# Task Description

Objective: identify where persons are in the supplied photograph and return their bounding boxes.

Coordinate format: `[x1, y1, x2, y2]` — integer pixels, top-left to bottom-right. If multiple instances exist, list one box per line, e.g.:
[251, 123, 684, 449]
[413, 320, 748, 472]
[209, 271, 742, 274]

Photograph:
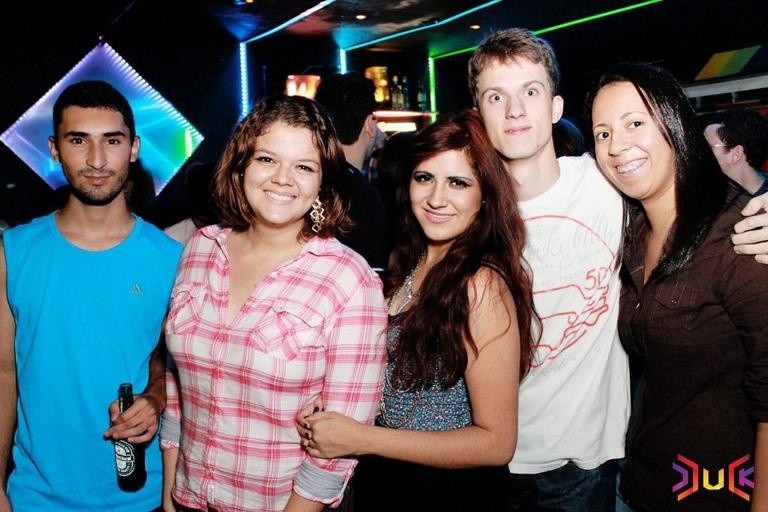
[292, 108, 545, 512]
[159, 93, 390, 512]
[465, 27, 768, 512]
[580, 58, 768, 512]
[319, 71, 394, 273]
[0, 79, 185, 512]
[699, 103, 768, 199]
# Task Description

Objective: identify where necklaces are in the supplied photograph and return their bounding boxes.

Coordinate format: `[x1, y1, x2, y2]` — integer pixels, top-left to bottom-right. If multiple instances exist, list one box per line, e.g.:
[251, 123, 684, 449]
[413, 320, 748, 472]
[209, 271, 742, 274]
[393, 254, 425, 313]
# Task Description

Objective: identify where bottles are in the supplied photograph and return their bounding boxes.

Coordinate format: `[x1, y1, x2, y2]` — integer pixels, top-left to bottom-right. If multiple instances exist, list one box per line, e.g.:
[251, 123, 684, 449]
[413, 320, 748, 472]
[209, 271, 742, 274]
[390, 74, 410, 109]
[113, 382, 147, 493]
[416, 79, 427, 111]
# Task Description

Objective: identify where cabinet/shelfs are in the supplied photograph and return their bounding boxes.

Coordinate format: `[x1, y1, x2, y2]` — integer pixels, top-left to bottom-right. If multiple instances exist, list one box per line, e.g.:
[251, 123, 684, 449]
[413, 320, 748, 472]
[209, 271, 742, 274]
[371, 110, 441, 138]
[680, 73, 768, 127]
